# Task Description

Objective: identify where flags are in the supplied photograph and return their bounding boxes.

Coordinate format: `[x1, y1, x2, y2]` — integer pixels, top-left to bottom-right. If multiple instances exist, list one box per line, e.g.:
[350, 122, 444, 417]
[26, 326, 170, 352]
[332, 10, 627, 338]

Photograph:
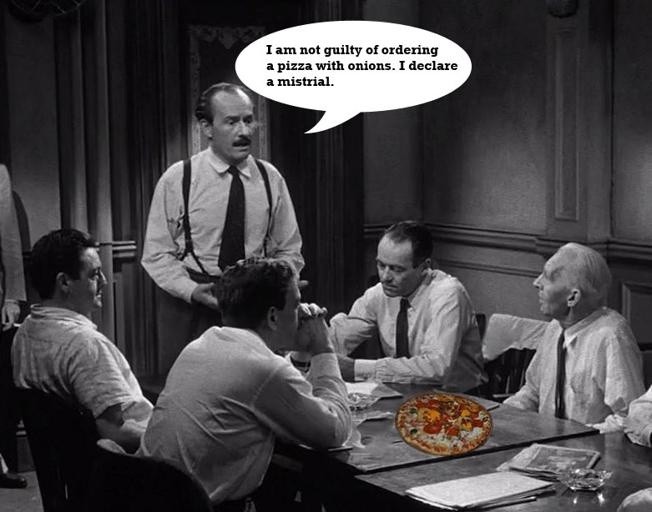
[4, 321, 24, 328]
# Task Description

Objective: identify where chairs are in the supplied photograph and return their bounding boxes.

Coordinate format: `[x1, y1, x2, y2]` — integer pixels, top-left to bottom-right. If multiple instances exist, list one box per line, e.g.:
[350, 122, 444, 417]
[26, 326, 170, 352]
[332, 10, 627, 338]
[17, 388, 213, 510]
[483, 313, 551, 400]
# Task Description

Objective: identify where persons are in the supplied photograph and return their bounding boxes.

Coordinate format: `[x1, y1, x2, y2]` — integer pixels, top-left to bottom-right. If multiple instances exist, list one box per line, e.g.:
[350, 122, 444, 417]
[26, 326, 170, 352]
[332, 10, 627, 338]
[498, 234, 633, 436]
[0, 158, 35, 490]
[326, 218, 491, 396]
[9, 226, 158, 457]
[621, 382, 651, 448]
[136, 80, 304, 384]
[125, 248, 353, 505]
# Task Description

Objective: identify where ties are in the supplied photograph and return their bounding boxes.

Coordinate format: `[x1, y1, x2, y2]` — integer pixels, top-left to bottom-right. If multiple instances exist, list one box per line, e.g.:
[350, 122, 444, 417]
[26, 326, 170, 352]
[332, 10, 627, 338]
[555, 334, 566, 418]
[394, 298, 411, 358]
[217, 166, 246, 272]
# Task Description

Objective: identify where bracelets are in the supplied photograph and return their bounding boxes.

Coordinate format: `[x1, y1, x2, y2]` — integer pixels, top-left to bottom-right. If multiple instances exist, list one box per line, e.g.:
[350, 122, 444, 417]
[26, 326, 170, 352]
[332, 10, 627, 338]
[289, 354, 313, 371]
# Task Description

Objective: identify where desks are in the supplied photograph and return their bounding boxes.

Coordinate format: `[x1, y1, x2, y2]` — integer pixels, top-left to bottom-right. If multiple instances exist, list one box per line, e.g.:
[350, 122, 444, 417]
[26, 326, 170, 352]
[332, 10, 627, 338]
[332, 383, 652, 511]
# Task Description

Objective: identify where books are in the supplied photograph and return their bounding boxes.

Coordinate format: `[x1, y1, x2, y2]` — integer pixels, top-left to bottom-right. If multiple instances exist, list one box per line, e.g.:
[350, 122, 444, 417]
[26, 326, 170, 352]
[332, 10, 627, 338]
[403, 440, 603, 509]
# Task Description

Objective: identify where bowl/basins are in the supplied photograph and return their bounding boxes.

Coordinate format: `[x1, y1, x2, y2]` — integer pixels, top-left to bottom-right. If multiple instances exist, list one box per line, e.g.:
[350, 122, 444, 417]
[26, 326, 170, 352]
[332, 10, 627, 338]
[557, 468, 614, 492]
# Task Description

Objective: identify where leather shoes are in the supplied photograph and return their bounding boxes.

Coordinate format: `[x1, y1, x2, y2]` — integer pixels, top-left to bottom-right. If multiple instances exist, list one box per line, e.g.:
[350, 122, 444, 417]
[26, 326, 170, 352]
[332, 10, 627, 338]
[0, 471, 27, 488]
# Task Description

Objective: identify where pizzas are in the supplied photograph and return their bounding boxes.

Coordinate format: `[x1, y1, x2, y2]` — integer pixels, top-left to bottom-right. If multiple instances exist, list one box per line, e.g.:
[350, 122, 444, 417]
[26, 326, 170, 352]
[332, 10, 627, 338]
[397, 392, 492, 455]
